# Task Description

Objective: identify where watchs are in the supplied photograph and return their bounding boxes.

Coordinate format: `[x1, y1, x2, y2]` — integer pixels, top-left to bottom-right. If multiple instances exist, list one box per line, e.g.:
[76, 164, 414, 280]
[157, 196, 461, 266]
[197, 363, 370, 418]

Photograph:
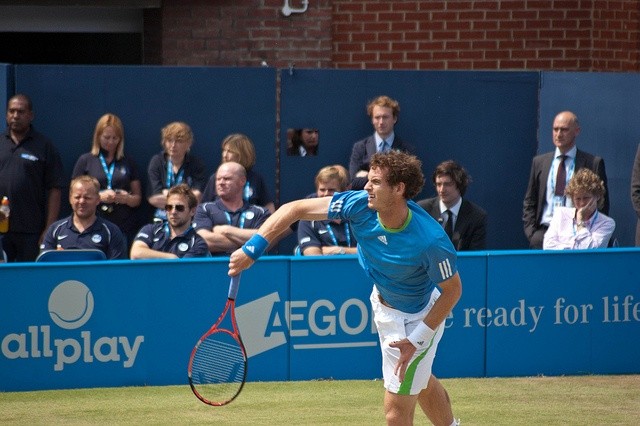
[338, 246, 346, 255]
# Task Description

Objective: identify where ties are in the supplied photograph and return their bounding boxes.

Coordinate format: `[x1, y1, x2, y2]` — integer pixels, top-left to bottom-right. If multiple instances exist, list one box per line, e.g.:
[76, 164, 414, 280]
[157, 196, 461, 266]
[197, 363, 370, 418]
[444, 209, 454, 242]
[379, 140, 388, 152]
[554, 154, 570, 197]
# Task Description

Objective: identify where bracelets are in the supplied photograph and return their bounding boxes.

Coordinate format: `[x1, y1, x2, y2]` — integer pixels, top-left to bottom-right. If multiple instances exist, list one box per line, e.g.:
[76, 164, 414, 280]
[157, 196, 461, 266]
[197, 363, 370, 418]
[406, 319, 437, 352]
[240, 232, 270, 263]
[577, 220, 591, 226]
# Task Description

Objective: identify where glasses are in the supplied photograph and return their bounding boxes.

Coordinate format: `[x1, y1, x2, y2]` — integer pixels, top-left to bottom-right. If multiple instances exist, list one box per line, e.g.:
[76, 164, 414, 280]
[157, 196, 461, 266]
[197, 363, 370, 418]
[165, 205, 186, 212]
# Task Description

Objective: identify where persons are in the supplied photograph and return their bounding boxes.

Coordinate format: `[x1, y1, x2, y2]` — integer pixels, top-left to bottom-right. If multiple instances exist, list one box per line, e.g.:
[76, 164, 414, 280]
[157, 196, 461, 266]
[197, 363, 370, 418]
[298, 163, 358, 257]
[190, 160, 274, 257]
[1, 92, 65, 261]
[630, 141, 639, 247]
[70, 112, 143, 257]
[520, 110, 611, 250]
[227, 148, 464, 426]
[146, 121, 208, 225]
[36, 174, 129, 262]
[127, 184, 213, 260]
[542, 167, 618, 250]
[414, 159, 489, 252]
[289, 127, 318, 156]
[198, 133, 277, 216]
[349, 95, 419, 191]
